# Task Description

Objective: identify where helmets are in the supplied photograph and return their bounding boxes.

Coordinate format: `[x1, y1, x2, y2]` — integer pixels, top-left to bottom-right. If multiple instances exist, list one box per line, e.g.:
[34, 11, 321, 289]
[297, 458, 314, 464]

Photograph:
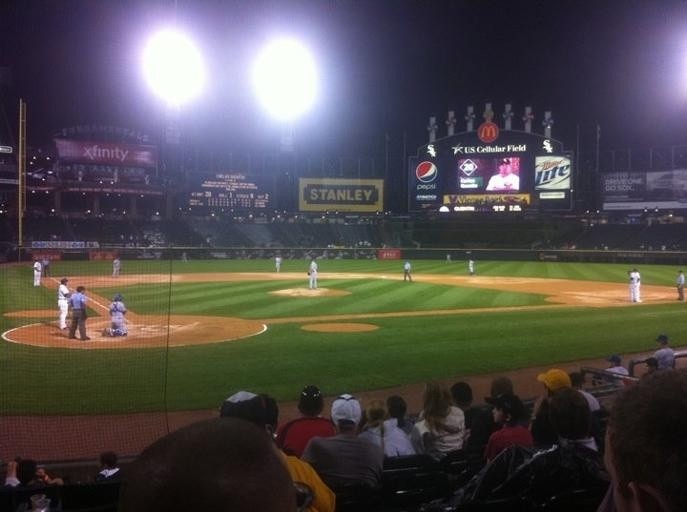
[331, 394, 361, 428]
[298, 384, 323, 416]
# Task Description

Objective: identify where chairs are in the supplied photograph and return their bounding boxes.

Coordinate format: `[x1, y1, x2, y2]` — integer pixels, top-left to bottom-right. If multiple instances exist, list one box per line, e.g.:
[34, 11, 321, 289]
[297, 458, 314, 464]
[277, 393, 618, 508]
[6, 455, 120, 504]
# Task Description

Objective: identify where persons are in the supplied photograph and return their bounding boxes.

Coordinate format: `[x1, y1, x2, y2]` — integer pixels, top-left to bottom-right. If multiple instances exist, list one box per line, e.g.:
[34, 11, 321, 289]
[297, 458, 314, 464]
[634, 268, 642, 302]
[484, 157, 520, 191]
[275, 254, 283, 274]
[33, 253, 128, 342]
[445, 253, 452, 265]
[628, 270, 635, 302]
[1, 332, 687, 511]
[468, 258, 475, 277]
[182, 251, 187, 264]
[307, 255, 318, 290]
[675, 270, 685, 301]
[403, 260, 412, 282]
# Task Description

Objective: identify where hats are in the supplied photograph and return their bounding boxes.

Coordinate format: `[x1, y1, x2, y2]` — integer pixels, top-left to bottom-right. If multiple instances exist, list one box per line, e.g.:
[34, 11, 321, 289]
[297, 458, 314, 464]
[655, 335, 668, 346]
[537, 368, 572, 393]
[485, 393, 522, 418]
[220, 390, 278, 432]
[605, 354, 622, 366]
